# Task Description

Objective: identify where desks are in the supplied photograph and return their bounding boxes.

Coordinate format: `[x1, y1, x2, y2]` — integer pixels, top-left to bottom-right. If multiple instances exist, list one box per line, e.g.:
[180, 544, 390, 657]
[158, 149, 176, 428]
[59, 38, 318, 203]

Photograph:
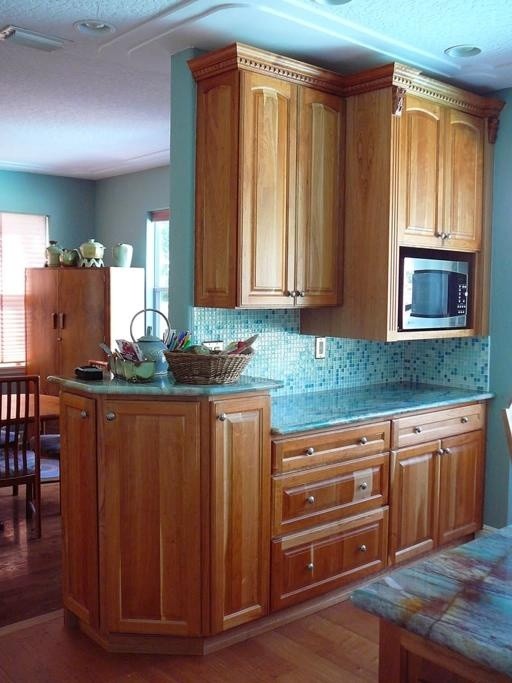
[0, 393, 60, 435]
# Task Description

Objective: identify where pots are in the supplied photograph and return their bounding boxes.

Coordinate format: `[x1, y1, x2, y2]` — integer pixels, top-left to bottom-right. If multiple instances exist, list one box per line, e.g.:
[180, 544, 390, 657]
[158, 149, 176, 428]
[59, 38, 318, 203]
[79, 238, 104, 259]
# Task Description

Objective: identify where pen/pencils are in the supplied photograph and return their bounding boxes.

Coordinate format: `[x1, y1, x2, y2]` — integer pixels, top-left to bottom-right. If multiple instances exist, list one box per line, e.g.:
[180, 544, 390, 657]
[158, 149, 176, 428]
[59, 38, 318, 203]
[169, 331, 191, 351]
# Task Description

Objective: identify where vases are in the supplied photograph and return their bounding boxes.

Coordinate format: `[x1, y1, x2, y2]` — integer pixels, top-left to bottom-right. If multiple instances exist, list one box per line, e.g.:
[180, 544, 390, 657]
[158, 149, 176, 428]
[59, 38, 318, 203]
[113, 243, 133, 267]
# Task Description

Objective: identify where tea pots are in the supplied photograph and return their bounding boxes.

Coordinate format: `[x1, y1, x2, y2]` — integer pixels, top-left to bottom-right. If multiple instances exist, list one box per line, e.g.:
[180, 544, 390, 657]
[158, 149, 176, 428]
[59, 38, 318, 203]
[129, 308, 171, 374]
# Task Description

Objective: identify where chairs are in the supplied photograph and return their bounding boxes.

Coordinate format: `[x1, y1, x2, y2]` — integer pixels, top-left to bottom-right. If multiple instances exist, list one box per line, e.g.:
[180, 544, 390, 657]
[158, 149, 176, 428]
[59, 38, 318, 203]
[0, 376, 60, 539]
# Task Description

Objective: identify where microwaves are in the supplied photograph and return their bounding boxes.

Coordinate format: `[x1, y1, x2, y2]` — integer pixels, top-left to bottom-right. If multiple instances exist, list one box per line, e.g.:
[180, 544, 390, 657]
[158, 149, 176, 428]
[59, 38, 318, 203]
[401, 257, 470, 329]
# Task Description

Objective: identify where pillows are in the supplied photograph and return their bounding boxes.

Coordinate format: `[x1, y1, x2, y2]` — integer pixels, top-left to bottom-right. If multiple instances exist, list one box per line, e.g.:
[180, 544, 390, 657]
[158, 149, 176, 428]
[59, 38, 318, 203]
[0, 448, 35, 478]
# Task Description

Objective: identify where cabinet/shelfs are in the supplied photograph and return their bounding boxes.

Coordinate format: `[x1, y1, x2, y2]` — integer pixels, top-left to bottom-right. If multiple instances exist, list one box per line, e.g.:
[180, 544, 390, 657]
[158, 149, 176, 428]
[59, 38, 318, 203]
[204, 391, 265, 657]
[391, 400, 488, 576]
[299, 62, 506, 343]
[187, 42, 345, 310]
[272, 419, 390, 629]
[57, 391, 204, 657]
[24, 267, 145, 398]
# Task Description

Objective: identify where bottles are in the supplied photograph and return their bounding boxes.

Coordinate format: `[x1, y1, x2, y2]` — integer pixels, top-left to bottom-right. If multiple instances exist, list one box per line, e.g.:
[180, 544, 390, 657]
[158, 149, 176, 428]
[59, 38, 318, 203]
[45, 240, 64, 267]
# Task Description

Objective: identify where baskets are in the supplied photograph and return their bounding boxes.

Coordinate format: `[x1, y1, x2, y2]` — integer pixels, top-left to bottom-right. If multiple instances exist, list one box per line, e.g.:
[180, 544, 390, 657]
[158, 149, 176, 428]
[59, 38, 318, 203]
[165, 346, 255, 384]
